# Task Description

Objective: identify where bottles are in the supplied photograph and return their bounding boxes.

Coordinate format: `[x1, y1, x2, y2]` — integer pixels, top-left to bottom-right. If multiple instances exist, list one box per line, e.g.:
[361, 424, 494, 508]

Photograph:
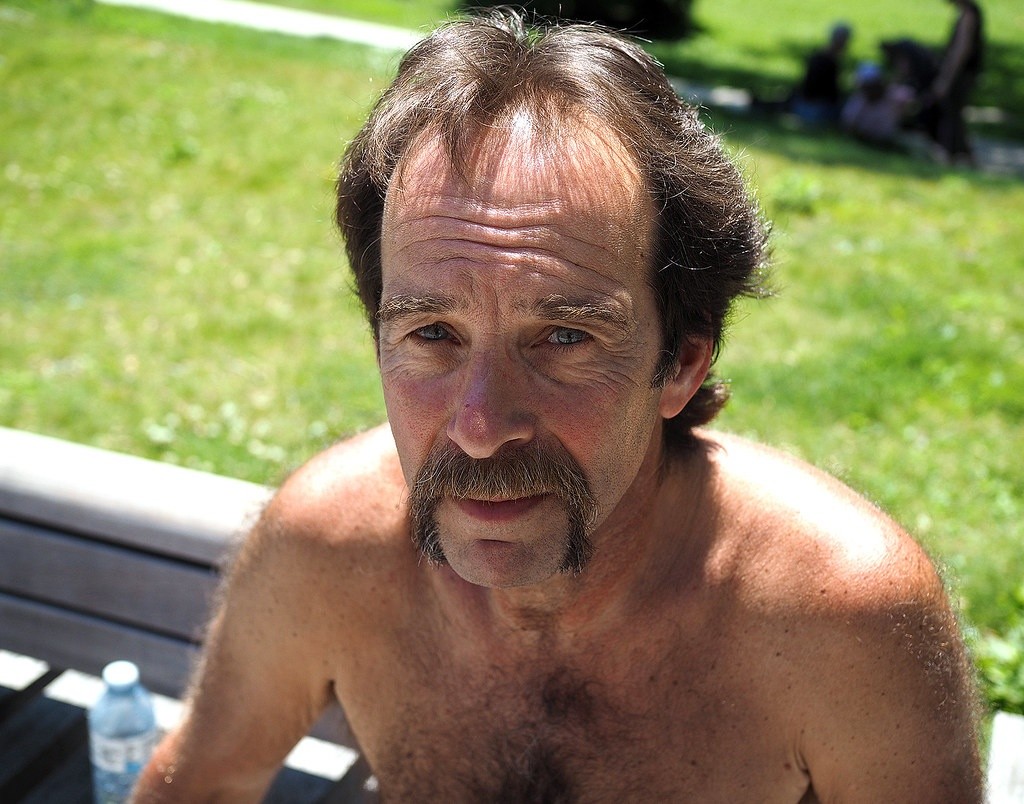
[87, 660, 158, 804]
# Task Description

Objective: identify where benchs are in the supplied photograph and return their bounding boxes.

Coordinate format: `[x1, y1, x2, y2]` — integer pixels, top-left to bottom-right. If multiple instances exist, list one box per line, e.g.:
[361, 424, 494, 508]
[0, 424, 384, 804]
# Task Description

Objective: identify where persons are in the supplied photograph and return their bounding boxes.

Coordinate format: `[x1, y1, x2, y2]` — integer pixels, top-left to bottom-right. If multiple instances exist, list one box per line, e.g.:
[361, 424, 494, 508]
[847, 36, 928, 150]
[928, 0, 986, 169]
[793, 25, 855, 137]
[120, 15, 990, 804]
